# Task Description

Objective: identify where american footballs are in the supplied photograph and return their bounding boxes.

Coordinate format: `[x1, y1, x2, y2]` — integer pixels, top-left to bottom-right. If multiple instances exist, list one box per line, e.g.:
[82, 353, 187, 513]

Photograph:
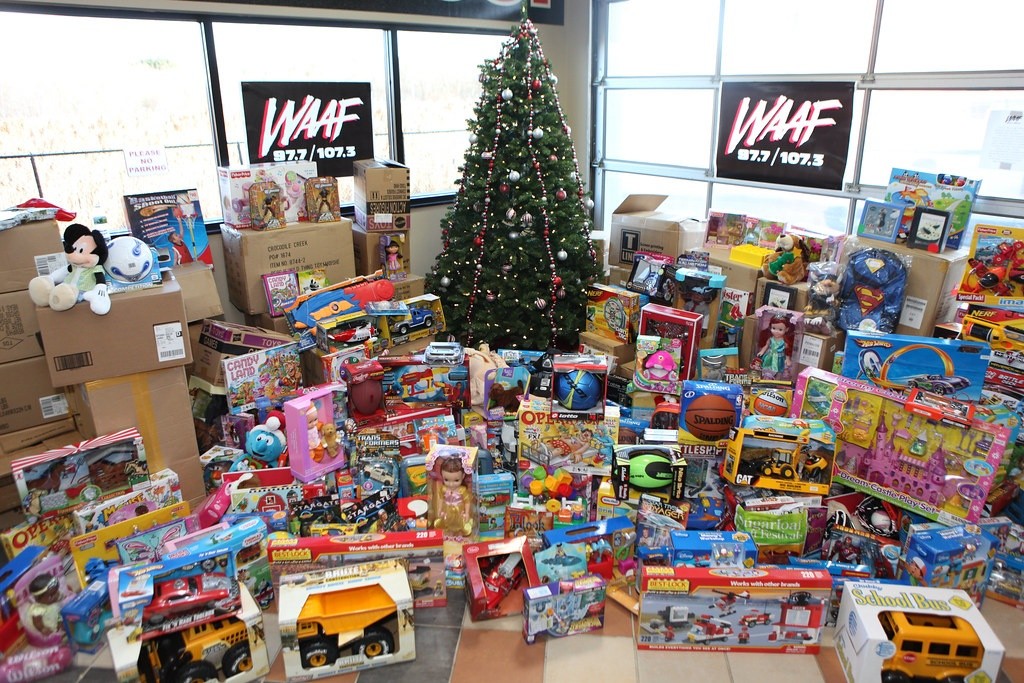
[350, 378, 382, 415]
[614, 456, 674, 489]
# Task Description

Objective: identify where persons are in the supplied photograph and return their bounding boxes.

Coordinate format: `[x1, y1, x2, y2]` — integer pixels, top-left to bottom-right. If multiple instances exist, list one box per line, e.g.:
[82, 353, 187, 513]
[756, 316, 792, 373]
[168, 215, 193, 266]
[384, 240, 403, 277]
[262, 194, 276, 218]
[684, 284, 710, 338]
[315, 187, 330, 212]
[307, 401, 325, 464]
[638, 529, 653, 546]
[46, 458, 68, 494]
[829, 537, 860, 565]
[29, 573, 67, 635]
[434, 458, 473, 535]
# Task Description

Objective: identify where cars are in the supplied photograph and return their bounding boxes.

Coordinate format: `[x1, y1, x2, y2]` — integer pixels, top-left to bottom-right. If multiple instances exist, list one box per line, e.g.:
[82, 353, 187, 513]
[390, 305, 436, 334]
[142, 572, 228, 625]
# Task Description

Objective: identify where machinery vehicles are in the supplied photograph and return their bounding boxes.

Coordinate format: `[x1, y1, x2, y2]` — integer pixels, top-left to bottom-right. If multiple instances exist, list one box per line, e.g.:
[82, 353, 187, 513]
[739, 445, 828, 484]
[137, 615, 253, 683]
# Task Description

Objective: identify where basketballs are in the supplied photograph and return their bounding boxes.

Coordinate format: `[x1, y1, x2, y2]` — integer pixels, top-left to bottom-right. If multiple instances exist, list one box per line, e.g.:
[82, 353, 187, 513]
[753, 391, 788, 417]
[685, 395, 736, 442]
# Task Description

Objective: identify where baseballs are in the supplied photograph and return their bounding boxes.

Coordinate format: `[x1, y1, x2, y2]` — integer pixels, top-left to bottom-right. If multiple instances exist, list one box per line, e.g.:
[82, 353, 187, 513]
[871, 510, 890, 530]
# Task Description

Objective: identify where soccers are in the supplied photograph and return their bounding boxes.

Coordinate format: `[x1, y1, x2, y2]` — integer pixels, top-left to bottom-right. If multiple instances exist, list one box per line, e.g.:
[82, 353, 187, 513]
[555, 370, 602, 411]
[98, 236, 154, 282]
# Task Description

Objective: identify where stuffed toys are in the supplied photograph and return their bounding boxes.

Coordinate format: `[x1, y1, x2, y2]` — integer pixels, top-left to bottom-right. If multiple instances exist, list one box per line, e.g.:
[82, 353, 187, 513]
[321, 424, 340, 457]
[205, 410, 287, 494]
[762, 234, 811, 284]
[803, 274, 840, 334]
[28, 222, 111, 315]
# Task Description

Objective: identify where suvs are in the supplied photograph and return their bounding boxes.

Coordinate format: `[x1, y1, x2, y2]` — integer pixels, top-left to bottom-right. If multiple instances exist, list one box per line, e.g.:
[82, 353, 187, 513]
[879, 611, 984, 683]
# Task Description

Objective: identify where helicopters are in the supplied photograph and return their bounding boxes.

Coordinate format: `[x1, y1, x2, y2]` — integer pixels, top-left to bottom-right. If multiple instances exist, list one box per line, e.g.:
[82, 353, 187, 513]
[327, 319, 383, 347]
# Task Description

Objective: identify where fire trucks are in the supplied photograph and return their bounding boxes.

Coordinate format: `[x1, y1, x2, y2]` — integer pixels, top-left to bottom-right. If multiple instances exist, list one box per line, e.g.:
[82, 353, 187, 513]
[477, 552, 526, 611]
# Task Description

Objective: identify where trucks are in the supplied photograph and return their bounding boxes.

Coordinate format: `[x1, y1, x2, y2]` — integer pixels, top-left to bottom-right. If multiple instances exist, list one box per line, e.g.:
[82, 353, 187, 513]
[296, 584, 397, 669]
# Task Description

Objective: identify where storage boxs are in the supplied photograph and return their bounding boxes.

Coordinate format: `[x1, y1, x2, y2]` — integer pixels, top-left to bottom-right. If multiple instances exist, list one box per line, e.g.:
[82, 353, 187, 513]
[1, 158, 1024, 683]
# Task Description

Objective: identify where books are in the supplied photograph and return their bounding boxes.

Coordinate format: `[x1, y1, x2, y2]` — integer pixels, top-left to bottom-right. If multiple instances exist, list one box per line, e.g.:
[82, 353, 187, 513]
[366, 301, 408, 315]
[260, 269, 330, 317]
